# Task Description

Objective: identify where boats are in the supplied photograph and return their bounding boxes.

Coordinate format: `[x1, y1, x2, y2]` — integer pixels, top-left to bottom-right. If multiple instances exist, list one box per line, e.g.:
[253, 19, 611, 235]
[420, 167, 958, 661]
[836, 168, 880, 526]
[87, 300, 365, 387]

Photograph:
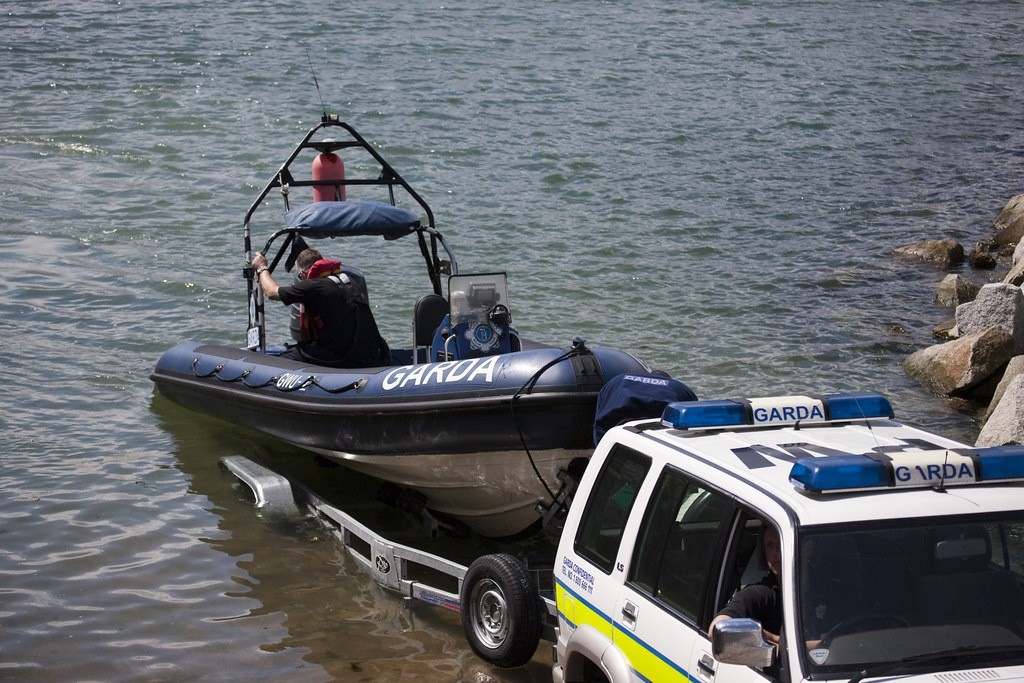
[151, 112, 699, 543]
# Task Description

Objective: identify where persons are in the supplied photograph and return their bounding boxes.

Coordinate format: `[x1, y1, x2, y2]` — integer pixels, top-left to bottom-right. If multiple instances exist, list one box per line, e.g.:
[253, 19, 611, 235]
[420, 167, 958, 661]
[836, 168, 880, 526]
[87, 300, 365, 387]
[708, 520, 861, 658]
[251, 249, 392, 369]
[645, 477, 704, 598]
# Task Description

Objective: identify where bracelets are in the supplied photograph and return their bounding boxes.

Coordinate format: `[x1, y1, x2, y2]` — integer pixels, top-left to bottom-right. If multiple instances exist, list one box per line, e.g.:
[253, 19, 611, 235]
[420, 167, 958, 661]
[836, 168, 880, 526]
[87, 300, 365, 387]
[256, 265, 269, 277]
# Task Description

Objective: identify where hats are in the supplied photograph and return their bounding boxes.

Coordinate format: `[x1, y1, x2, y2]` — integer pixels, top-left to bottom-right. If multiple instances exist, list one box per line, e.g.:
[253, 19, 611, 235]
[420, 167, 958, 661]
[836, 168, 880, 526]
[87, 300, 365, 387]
[285, 235, 310, 273]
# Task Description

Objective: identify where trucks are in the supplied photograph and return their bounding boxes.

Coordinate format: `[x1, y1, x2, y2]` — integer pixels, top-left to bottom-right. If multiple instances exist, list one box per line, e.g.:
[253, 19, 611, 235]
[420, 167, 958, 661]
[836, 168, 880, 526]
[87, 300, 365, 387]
[217, 391, 1023, 682]
